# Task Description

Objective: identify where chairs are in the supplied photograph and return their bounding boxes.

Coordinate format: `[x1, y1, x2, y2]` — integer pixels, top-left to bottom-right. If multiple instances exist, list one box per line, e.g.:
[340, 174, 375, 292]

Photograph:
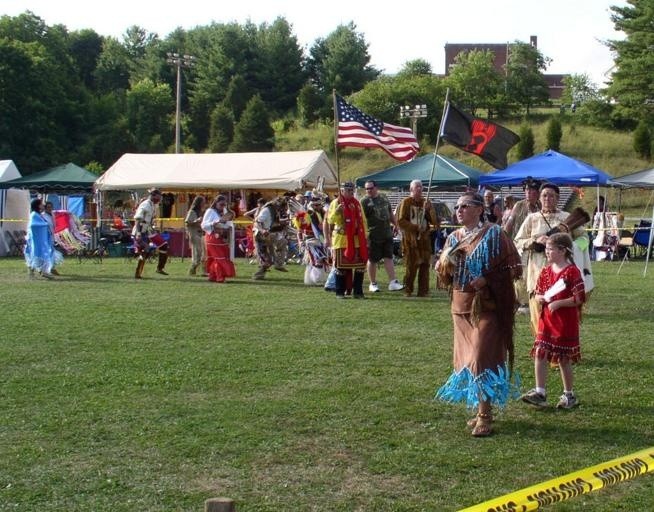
[6, 230, 27, 257]
[633, 219, 654, 259]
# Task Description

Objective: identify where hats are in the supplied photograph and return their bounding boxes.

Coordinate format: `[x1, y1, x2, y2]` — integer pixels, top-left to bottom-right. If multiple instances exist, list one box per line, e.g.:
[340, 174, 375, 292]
[339, 183, 355, 191]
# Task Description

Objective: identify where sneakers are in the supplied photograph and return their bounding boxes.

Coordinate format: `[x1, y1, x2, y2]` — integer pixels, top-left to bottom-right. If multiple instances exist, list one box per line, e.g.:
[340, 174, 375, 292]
[336, 294, 345, 299]
[556, 392, 578, 409]
[155, 270, 168, 275]
[276, 268, 289, 273]
[522, 392, 547, 407]
[388, 279, 405, 291]
[369, 283, 380, 293]
[353, 295, 368, 300]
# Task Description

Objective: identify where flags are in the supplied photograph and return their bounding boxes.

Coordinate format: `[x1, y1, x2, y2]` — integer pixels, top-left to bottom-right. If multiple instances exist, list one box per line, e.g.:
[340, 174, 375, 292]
[336, 93, 420, 162]
[440, 101, 521, 171]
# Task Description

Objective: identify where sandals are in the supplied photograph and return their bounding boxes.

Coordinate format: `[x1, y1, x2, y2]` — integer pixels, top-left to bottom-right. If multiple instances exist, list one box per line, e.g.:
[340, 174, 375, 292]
[467, 414, 493, 437]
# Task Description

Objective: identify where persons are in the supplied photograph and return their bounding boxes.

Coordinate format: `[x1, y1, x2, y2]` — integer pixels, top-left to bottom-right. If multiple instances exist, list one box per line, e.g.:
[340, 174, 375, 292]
[595, 197, 609, 223]
[185, 181, 589, 335]
[435, 191, 523, 437]
[569, 101, 576, 114]
[25, 198, 64, 280]
[521, 233, 586, 409]
[131, 189, 169, 279]
[559, 103, 566, 113]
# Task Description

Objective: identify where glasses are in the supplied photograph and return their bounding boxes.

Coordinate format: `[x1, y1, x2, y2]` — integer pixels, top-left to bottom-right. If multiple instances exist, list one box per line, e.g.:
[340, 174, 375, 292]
[454, 204, 478, 209]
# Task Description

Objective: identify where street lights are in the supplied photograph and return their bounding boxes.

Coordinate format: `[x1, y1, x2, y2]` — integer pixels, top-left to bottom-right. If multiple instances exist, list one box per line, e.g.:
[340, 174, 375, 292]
[399, 104, 428, 160]
[165, 51, 195, 153]
